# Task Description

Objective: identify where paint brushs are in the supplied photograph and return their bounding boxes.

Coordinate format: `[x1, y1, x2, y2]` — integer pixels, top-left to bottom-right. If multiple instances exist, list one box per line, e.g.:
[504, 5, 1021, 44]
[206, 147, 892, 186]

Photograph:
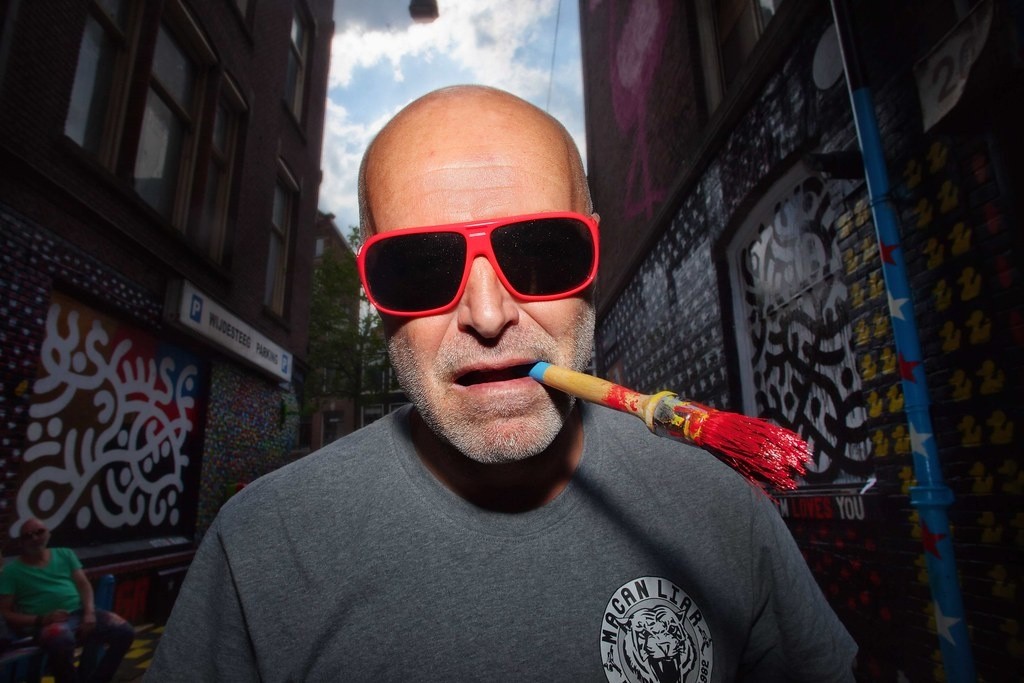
[529, 361, 814, 507]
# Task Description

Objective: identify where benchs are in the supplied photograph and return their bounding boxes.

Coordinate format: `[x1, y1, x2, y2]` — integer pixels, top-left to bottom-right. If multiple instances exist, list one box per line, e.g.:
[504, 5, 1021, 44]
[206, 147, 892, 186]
[0, 615, 107, 683]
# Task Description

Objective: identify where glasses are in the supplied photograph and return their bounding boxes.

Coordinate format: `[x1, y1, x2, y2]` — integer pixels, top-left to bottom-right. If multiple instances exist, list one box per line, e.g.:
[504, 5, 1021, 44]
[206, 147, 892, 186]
[22, 528, 46, 540]
[357, 210, 600, 317]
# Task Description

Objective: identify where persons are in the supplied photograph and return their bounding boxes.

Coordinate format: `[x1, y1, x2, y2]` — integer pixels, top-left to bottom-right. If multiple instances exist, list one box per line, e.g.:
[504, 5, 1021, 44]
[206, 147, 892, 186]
[147, 83, 859, 683]
[1, 521, 136, 683]
